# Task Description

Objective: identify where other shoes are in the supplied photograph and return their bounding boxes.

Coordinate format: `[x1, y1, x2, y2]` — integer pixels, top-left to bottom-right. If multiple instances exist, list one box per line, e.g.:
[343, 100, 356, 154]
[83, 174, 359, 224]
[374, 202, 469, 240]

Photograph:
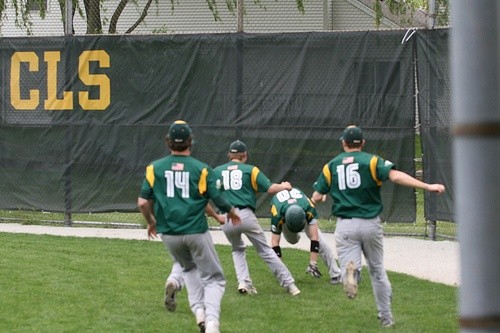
[343, 260, 359, 300]
[287, 284, 301, 296]
[237, 283, 257, 295]
[331, 276, 342, 284]
[164, 282, 177, 312]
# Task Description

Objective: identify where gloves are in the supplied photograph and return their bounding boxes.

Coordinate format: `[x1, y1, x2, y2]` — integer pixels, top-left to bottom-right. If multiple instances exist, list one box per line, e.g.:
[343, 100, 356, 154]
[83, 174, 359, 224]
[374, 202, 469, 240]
[304, 262, 323, 279]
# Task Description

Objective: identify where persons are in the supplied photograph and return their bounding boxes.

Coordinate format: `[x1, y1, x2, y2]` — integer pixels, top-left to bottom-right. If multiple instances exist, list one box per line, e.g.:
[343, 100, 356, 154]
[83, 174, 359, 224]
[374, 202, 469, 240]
[212, 140, 301, 296]
[270, 186, 342, 284]
[137, 120, 241, 333]
[310, 125, 446, 327]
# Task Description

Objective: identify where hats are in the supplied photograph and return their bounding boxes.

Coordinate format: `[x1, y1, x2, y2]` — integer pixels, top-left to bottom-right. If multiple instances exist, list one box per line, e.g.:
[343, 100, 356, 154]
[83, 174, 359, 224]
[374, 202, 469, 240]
[340, 125, 364, 144]
[285, 205, 306, 233]
[229, 140, 247, 153]
[167, 121, 192, 143]
